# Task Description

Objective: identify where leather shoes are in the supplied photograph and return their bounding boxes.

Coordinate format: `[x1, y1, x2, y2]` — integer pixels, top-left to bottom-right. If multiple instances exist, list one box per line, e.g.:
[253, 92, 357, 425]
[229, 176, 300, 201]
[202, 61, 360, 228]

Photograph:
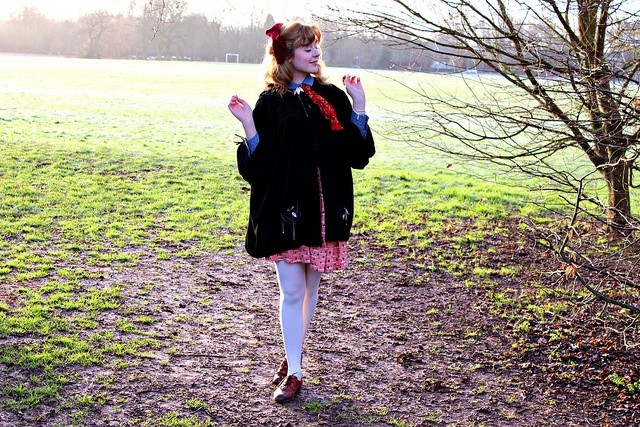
[272, 358, 287, 386]
[273, 375, 302, 402]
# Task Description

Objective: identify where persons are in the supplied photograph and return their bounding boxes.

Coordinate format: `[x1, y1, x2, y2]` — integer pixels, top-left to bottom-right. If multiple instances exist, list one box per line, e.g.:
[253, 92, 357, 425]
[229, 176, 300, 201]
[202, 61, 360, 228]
[227, 20, 376, 404]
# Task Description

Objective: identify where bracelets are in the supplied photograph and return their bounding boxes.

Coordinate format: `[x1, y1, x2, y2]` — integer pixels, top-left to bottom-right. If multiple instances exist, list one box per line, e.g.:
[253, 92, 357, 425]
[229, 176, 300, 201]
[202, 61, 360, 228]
[352, 109, 365, 122]
[356, 110, 366, 112]
